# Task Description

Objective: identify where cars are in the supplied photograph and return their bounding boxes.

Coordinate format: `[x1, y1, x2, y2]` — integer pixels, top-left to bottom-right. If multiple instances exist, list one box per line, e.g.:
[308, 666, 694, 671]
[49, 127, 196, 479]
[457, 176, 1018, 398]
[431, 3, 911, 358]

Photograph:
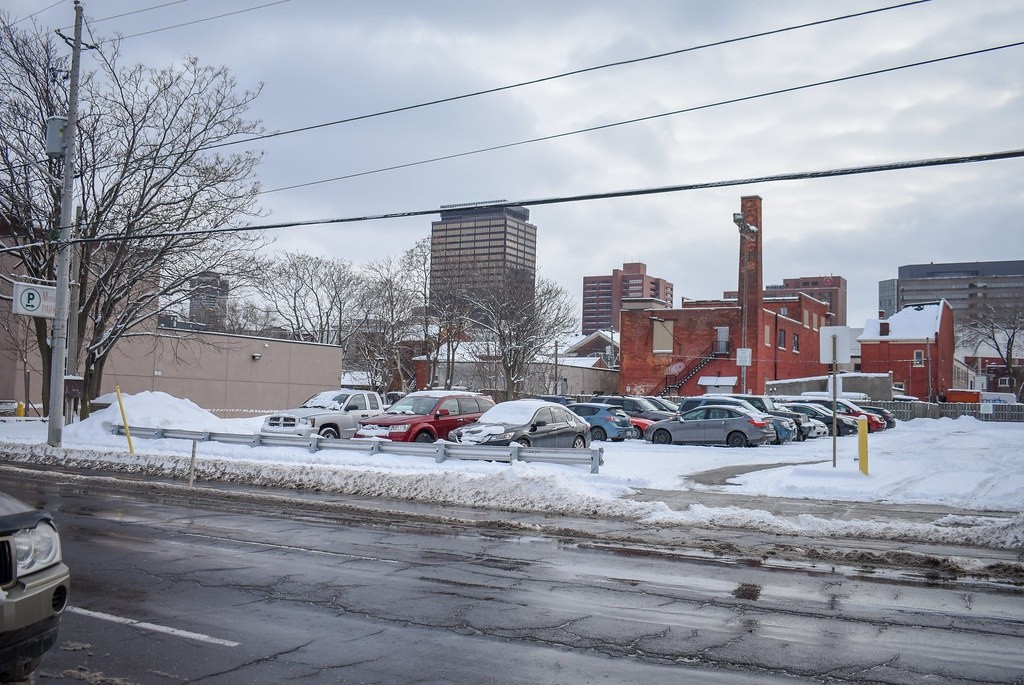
[643, 405, 776, 447]
[447, 399, 592, 451]
[625, 415, 654, 437]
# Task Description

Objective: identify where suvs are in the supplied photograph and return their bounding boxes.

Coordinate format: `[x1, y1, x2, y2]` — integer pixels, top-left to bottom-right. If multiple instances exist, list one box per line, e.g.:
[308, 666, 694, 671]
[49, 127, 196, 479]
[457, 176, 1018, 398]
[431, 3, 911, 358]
[0, 492, 72, 680]
[260, 388, 392, 441]
[587, 393, 676, 424]
[355, 391, 499, 442]
[642, 395, 683, 414]
[564, 404, 634, 441]
[677, 391, 895, 445]
